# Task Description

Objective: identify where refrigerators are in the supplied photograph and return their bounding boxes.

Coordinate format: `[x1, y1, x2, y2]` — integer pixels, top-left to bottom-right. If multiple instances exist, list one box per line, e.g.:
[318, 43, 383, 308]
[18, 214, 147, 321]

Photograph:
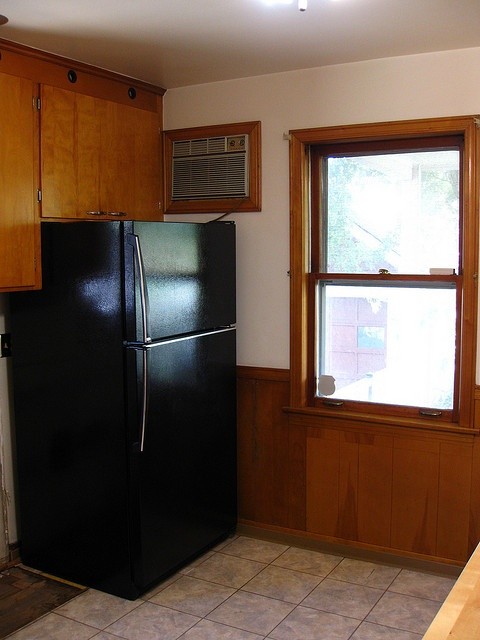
[0, 214, 238, 601]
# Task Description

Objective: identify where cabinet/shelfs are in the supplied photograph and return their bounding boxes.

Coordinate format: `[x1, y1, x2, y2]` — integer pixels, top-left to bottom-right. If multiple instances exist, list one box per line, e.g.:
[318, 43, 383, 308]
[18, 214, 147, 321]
[40, 82, 165, 221]
[0, 71, 43, 293]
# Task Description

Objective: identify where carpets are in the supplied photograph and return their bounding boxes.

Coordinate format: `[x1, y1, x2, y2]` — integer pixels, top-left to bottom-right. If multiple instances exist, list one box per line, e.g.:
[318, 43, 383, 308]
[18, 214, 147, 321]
[0, 566, 89, 640]
